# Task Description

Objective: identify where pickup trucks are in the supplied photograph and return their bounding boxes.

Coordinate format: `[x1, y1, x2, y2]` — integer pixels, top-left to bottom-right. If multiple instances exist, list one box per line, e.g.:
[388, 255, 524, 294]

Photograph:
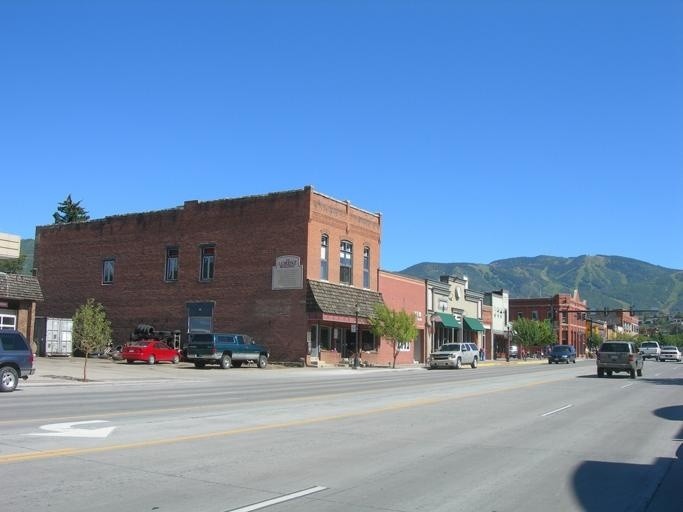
[638, 341, 661, 361]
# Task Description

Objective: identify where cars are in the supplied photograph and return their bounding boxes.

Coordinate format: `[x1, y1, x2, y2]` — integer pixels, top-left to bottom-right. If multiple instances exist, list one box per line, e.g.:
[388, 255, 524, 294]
[660, 346, 682, 362]
[122, 339, 182, 364]
[548, 344, 576, 364]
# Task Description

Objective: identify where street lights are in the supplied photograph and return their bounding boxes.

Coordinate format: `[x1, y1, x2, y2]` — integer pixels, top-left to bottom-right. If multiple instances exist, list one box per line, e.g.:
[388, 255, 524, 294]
[352, 304, 361, 369]
[507, 323, 510, 362]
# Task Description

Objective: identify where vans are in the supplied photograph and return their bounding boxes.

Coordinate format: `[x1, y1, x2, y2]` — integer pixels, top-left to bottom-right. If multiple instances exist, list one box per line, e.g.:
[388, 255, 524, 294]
[596, 341, 643, 378]
[0, 329, 36, 392]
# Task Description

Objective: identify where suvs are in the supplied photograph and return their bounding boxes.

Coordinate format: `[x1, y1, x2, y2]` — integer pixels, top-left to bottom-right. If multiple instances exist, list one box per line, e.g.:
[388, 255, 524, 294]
[187, 333, 271, 369]
[430, 342, 479, 369]
